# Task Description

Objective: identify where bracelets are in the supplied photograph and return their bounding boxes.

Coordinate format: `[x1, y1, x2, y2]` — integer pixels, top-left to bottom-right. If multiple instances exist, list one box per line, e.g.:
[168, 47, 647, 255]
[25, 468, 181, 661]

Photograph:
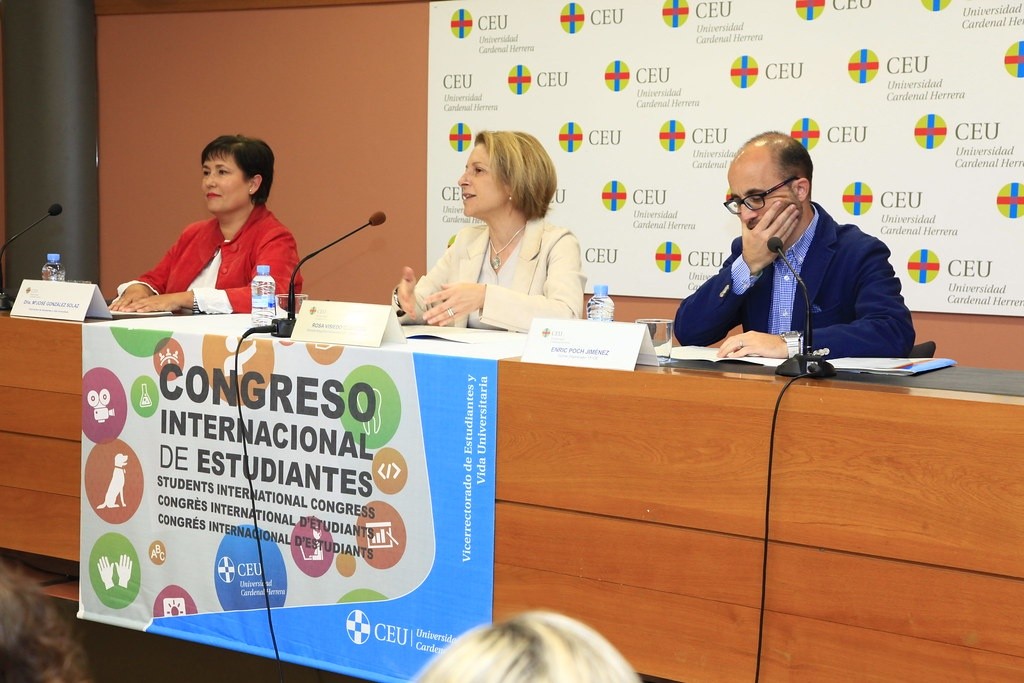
[192, 293, 200, 314]
[394, 289, 401, 308]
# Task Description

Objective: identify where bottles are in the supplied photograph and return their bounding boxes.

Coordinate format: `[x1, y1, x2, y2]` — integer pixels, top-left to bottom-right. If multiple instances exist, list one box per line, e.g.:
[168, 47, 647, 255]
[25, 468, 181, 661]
[585, 285, 617, 322]
[41, 253, 66, 282]
[250, 265, 280, 326]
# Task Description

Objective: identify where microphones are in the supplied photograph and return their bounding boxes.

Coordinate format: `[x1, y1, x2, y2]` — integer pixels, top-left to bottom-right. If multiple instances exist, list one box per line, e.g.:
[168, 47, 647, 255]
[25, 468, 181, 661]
[767, 237, 837, 377]
[0, 204, 62, 310]
[271, 211, 386, 336]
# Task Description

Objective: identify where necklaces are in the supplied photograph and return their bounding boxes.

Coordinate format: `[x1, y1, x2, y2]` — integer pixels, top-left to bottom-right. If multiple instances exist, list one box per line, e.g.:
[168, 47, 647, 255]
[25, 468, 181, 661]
[490, 224, 526, 270]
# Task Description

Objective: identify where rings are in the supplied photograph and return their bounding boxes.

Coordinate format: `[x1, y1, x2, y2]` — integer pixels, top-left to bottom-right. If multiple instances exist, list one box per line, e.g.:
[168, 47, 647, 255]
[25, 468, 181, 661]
[448, 309, 454, 317]
[740, 340, 743, 348]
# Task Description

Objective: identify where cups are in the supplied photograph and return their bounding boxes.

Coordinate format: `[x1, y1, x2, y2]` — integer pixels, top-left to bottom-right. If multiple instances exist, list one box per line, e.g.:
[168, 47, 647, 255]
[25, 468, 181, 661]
[634, 319, 674, 366]
[275, 293, 308, 318]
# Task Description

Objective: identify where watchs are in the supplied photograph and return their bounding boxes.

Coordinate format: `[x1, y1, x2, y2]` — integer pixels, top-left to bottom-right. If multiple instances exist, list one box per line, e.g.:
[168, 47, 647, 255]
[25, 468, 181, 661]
[781, 331, 800, 359]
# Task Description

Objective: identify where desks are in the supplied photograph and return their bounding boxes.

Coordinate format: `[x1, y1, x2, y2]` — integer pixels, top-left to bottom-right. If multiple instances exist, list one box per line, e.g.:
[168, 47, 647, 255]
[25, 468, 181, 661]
[0, 309, 1024, 683]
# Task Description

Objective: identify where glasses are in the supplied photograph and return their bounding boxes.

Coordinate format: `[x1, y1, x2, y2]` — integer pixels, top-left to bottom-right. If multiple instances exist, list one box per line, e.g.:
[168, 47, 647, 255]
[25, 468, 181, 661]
[723, 176, 799, 214]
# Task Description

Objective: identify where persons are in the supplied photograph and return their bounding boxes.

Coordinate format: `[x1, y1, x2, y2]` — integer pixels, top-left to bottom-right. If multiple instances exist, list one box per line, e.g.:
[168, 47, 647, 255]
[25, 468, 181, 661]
[412, 610, 644, 683]
[673, 130, 916, 361]
[0, 560, 97, 683]
[108, 135, 303, 315]
[392, 130, 587, 334]
